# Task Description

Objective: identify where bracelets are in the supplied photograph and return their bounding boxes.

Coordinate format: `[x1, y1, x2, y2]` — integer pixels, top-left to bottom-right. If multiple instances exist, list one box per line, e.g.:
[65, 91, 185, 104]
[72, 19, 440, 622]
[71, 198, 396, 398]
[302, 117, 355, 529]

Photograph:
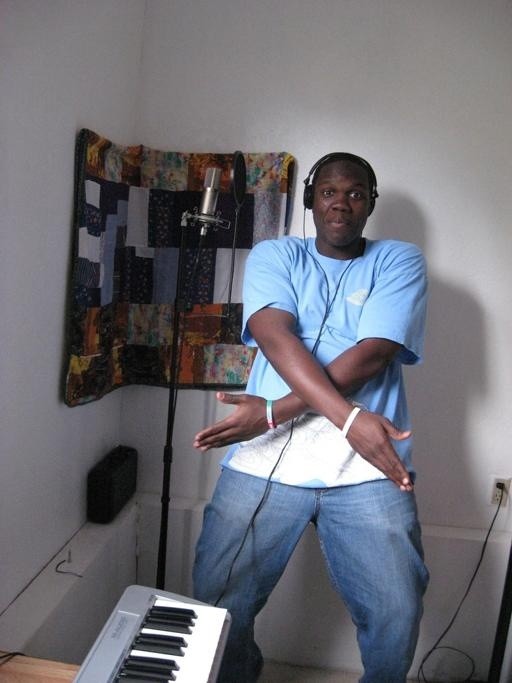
[341, 408, 361, 441]
[265, 400, 275, 430]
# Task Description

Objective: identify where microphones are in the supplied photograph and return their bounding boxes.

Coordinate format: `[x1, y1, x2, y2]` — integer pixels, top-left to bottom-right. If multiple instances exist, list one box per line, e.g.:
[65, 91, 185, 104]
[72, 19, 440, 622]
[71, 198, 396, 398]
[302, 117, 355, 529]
[199, 166, 222, 237]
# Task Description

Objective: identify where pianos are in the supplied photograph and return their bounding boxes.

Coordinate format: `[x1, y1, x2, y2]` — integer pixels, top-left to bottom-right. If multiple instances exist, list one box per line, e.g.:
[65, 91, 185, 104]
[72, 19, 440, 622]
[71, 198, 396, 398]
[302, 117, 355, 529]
[72, 584, 233, 683]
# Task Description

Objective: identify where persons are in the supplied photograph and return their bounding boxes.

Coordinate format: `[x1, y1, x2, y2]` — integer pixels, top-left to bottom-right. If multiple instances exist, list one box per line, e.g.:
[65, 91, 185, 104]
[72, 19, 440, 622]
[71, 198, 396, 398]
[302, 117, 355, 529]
[192, 152, 430, 683]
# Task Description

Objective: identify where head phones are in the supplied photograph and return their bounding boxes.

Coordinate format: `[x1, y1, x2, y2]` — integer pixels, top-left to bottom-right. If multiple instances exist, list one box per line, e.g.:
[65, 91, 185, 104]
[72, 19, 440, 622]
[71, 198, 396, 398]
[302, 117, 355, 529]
[304, 152, 379, 216]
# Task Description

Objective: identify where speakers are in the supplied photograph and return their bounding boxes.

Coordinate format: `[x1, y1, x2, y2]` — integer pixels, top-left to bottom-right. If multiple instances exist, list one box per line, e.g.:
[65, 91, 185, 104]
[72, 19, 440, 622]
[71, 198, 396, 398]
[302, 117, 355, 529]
[86, 445, 137, 525]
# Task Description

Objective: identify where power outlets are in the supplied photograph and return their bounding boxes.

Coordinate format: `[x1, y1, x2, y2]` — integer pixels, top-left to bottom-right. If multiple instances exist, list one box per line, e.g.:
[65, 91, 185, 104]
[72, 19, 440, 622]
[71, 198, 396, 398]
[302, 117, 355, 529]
[491, 478, 511, 507]
